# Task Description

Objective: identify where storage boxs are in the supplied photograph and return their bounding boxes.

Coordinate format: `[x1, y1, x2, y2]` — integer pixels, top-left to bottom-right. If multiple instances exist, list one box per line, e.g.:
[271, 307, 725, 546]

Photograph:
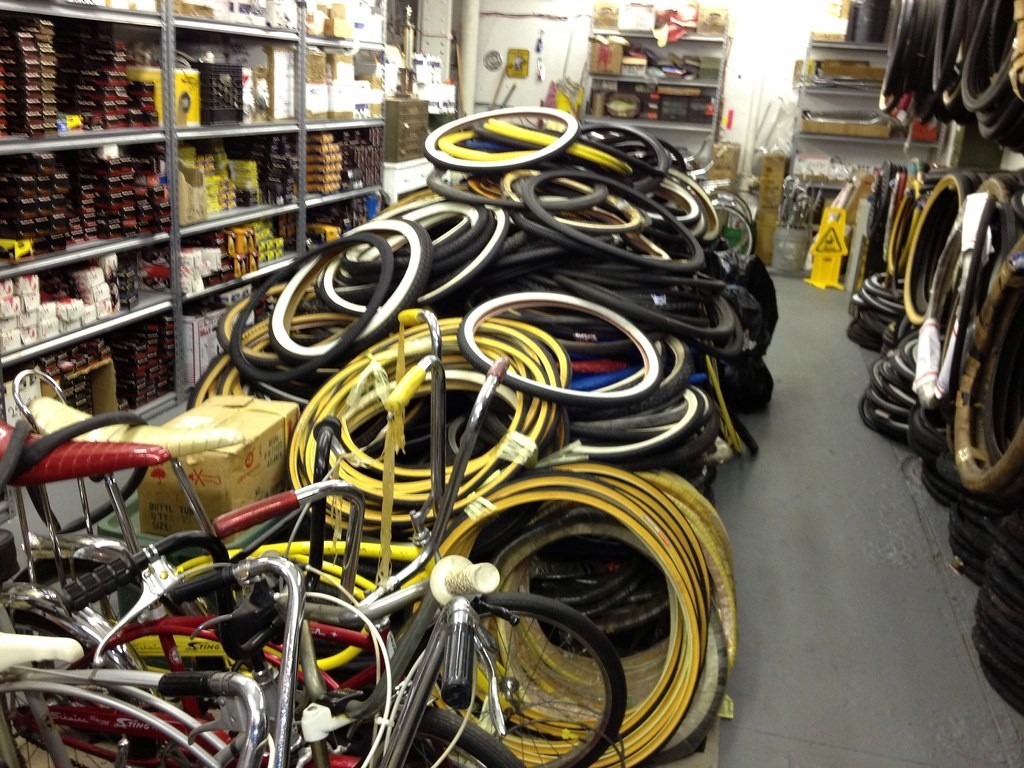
[621, 56, 648, 77]
[643, 94, 716, 124]
[698, 9, 729, 36]
[589, 40, 623, 75]
[844, 171, 875, 225]
[138, 395, 301, 544]
[126, 66, 201, 128]
[1, 17, 159, 136]
[67, 0, 351, 39]
[99, 494, 290, 618]
[224, 40, 384, 124]
[0, 191, 382, 352]
[811, 0, 853, 42]
[617, 5, 657, 32]
[755, 156, 790, 256]
[0, 126, 385, 264]
[0, 284, 255, 432]
[708, 144, 740, 227]
[192, 63, 243, 123]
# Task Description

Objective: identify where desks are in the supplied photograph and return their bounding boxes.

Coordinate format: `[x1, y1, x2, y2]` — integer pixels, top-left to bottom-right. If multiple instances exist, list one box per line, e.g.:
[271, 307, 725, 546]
[381, 156, 451, 207]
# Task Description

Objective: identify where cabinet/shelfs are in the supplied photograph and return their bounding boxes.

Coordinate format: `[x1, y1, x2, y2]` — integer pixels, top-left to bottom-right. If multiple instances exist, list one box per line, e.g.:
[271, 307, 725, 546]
[0, 0, 386, 425]
[584, 28, 732, 169]
[781, 41, 957, 232]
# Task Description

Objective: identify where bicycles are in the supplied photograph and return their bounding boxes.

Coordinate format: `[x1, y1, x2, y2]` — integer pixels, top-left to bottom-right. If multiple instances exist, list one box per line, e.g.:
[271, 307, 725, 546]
[1, 310, 628, 768]
